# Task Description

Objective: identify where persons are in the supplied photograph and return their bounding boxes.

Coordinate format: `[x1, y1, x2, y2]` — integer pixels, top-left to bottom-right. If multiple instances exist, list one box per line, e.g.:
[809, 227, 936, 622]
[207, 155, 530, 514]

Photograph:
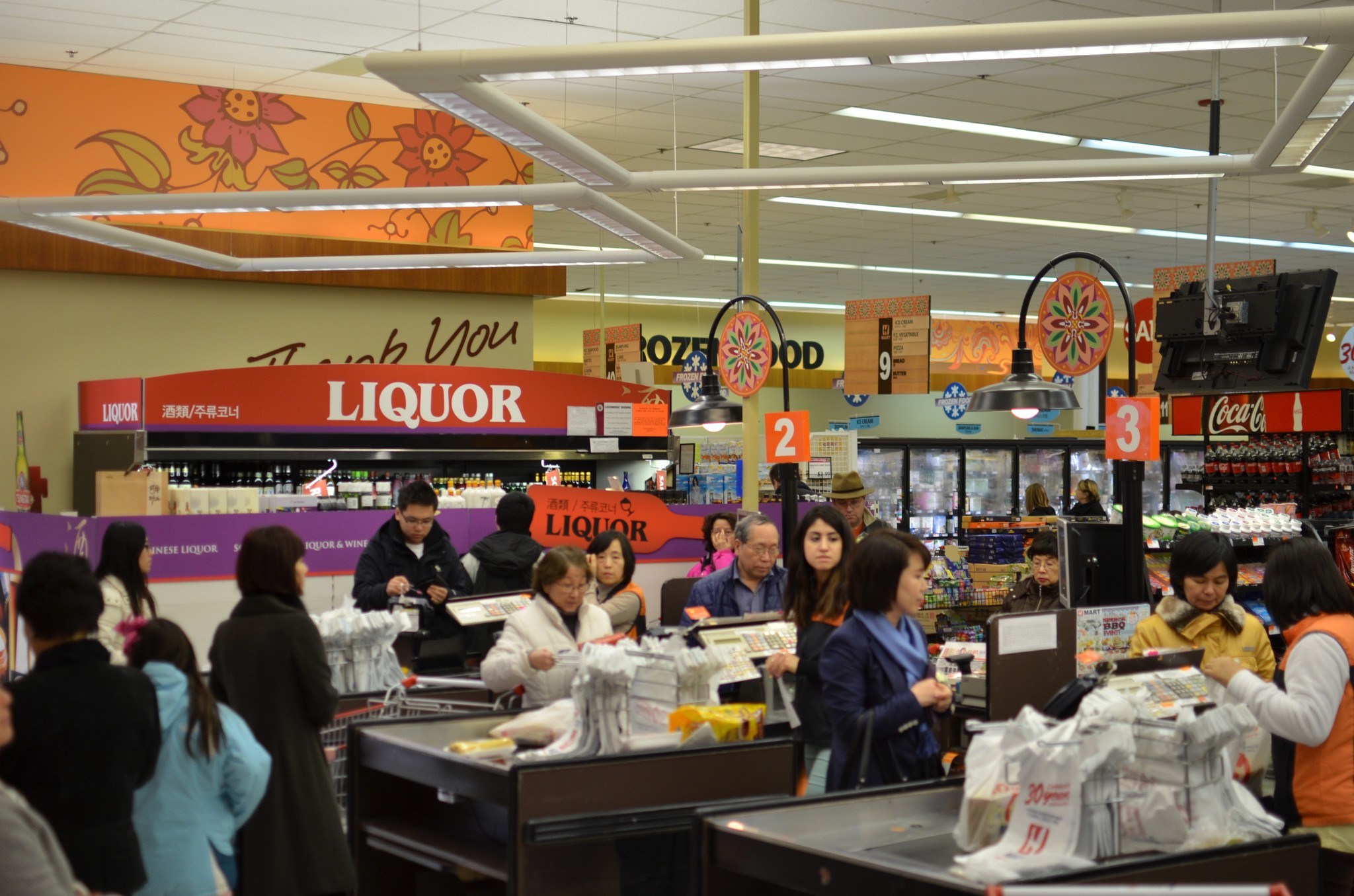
[0, 689, 90, 896]
[1068, 479, 1108, 521]
[480, 545, 614, 708]
[648, 478, 655, 490]
[1025, 483, 1056, 516]
[457, 492, 545, 655]
[1205, 540, 1354, 896]
[586, 530, 646, 643]
[1130, 530, 1277, 680]
[124, 617, 272, 896]
[769, 463, 821, 496]
[818, 530, 953, 791]
[87, 522, 157, 666]
[821, 471, 893, 544]
[207, 524, 361, 896]
[686, 512, 738, 577]
[682, 514, 788, 629]
[691, 475, 700, 504]
[766, 505, 856, 795]
[0, 550, 163, 896]
[994, 530, 1065, 616]
[352, 479, 474, 656]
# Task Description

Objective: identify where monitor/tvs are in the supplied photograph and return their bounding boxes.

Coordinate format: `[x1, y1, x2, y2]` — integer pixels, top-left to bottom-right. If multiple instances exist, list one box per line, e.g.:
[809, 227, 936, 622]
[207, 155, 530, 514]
[1042, 646, 1207, 716]
[1153, 268, 1338, 393]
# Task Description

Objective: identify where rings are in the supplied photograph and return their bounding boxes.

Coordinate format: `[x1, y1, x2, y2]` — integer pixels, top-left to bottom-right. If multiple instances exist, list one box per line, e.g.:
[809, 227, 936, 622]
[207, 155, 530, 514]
[400, 582, 403, 586]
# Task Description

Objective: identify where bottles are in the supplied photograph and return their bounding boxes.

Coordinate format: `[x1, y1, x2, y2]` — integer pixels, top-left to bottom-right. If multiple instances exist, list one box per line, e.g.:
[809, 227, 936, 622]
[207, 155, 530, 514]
[1180, 435, 1354, 519]
[622, 471, 630, 491]
[144, 458, 592, 514]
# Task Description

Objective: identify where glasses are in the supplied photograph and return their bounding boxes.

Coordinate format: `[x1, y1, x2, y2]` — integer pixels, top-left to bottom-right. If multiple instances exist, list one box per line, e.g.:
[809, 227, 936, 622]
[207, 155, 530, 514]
[1029, 560, 1059, 571]
[743, 541, 780, 557]
[400, 512, 435, 527]
[555, 580, 590, 594]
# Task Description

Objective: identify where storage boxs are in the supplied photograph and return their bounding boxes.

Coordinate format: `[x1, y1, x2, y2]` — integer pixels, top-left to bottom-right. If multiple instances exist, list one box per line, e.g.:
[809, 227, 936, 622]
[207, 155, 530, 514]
[676, 440, 744, 507]
[960, 513, 1107, 589]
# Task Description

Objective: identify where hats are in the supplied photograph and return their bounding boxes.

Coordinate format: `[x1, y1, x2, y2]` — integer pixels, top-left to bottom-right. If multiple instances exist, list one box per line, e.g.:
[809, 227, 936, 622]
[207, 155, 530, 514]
[823, 471, 875, 499]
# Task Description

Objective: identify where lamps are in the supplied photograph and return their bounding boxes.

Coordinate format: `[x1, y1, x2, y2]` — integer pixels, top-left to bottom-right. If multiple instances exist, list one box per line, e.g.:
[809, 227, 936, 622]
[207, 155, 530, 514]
[667, 293, 791, 433]
[965, 251, 1136, 419]
